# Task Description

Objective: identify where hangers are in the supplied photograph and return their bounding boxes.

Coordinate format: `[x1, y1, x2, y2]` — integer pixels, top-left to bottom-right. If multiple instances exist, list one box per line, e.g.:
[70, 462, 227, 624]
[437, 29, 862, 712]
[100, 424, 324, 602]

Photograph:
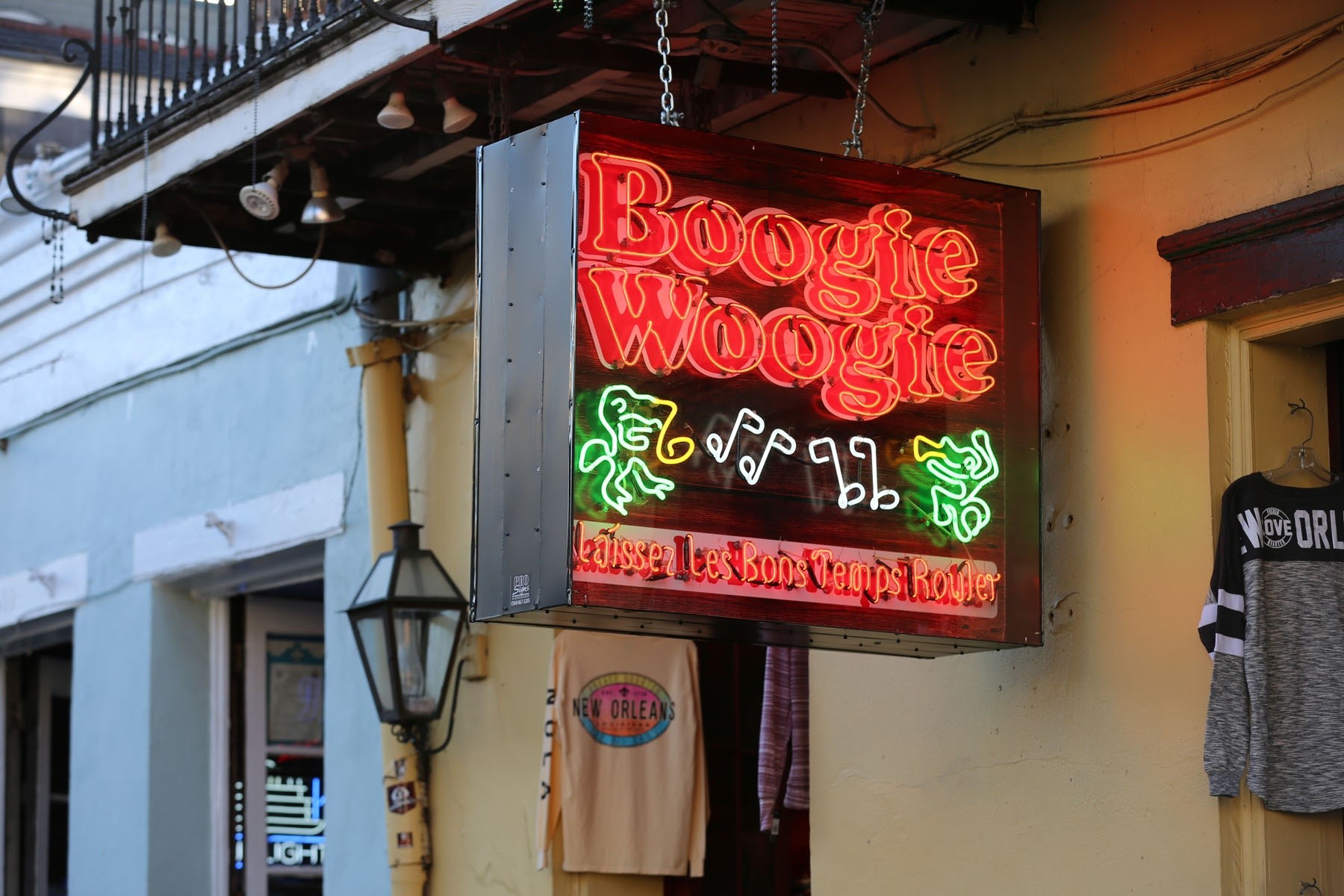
[1223, 407, 1344, 503]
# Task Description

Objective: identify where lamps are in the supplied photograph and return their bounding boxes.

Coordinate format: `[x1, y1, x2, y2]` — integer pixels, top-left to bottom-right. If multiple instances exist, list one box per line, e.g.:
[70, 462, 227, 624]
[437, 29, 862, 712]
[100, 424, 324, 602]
[432, 79, 477, 134]
[239, 160, 289, 220]
[300, 166, 345, 224]
[150, 222, 182, 256]
[377, 73, 414, 129]
[339, 522, 489, 778]
